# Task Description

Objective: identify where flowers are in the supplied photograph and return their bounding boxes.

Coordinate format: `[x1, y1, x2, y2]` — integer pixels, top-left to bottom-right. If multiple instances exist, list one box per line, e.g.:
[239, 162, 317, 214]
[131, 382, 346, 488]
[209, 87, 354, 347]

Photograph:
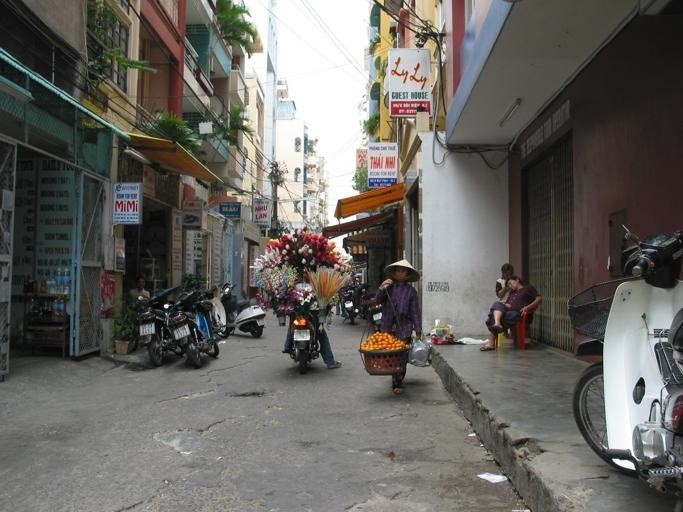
[248, 226, 341, 316]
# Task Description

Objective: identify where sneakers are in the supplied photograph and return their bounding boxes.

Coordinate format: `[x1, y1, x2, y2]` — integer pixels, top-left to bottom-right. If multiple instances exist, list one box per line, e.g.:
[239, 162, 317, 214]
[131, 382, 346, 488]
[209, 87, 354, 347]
[285, 345, 292, 352]
[327, 360, 341, 368]
[394, 386, 404, 393]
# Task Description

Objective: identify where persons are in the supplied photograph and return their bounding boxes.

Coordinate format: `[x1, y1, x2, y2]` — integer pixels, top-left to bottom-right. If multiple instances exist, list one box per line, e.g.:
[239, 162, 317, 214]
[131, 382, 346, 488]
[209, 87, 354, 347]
[496, 264, 514, 304]
[282, 312, 342, 369]
[129, 276, 150, 299]
[375, 258, 422, 395]
[479, 276, 542, 351]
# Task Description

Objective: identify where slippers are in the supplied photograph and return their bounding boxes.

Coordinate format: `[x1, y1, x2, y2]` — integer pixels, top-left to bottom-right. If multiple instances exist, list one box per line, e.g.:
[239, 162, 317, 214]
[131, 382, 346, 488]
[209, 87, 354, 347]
[491, 324, 503, 332]
[481, 345, 496, 350]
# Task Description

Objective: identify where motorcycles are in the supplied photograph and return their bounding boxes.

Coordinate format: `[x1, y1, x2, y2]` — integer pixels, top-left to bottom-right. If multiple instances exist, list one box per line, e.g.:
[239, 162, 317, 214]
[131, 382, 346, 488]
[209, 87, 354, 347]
[341, 284, 382, 327]
[289, 309, 320, 375]
[137, 281, 267, 366]
[564, 229, 682, 509]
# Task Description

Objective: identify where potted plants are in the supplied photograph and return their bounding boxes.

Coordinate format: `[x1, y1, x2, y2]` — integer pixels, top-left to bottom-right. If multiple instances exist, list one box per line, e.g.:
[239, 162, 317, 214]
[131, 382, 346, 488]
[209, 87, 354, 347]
[114, 295, 144, 354]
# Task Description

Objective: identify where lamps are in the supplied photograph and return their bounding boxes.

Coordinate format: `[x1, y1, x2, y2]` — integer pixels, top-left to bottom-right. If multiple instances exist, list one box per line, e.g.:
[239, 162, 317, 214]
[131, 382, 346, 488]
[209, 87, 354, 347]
[123, 145, 152, 166]
[499, 98, 521, 128]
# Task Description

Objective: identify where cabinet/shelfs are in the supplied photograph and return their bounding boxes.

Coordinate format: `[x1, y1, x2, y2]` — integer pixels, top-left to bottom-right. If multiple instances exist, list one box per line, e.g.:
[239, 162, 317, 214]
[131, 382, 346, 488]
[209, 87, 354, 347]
[23, 293, 70, 359]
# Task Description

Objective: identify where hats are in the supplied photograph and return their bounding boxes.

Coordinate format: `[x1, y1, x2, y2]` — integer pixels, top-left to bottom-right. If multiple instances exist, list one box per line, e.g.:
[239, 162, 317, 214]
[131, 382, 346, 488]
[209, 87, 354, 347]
[385, 260, 420, 282]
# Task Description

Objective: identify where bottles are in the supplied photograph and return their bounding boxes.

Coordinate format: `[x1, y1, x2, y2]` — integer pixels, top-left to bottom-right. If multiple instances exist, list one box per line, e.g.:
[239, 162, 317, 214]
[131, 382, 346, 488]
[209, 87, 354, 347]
[19, 264, 74, 321]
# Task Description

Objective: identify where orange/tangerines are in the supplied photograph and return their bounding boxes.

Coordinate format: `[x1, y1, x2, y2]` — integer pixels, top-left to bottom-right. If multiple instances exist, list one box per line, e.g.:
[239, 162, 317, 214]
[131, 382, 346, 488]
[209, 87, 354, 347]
[361, 331, 408, 351]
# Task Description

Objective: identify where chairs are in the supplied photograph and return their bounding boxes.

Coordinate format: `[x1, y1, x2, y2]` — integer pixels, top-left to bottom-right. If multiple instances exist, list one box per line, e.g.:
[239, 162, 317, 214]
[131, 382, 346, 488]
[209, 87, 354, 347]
[496, 310, 535, 351]
